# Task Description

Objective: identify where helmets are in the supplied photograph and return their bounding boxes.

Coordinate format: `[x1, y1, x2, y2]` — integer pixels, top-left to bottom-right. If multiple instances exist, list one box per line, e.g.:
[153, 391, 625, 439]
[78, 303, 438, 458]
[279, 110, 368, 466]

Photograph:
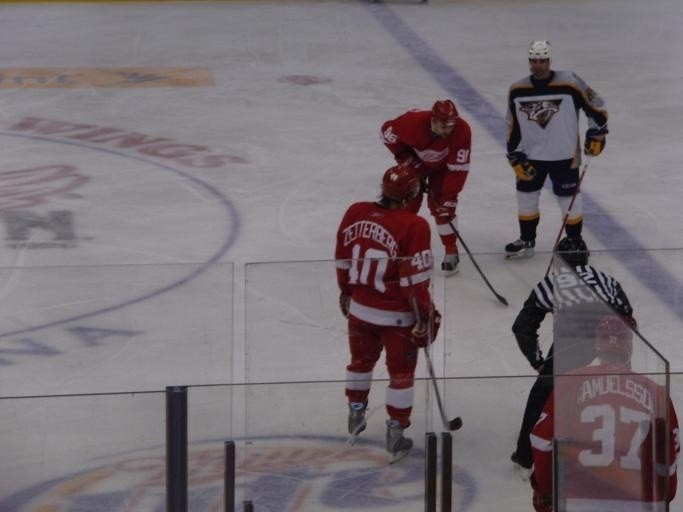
[527, 39, 553, 64]
[431, 99, 461, 127]
[594, 316, 635, 358]
[551, 235, 590, 266]
[382, 164, 421, 201]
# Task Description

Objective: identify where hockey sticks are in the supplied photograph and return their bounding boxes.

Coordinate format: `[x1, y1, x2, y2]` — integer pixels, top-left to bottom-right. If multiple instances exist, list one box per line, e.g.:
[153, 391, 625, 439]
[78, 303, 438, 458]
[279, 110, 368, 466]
[407, 292, 462, 431]
[432, 192, 509, 305]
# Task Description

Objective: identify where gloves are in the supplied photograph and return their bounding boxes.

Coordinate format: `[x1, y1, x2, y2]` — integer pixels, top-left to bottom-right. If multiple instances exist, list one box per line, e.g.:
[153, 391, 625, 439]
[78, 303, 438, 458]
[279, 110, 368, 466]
[511, 160, 538, 182]
[437, 199, 459, 222]
[336, 291, 353, 320]
[412, 310, 441, 348]
[582, 132, 606, 156]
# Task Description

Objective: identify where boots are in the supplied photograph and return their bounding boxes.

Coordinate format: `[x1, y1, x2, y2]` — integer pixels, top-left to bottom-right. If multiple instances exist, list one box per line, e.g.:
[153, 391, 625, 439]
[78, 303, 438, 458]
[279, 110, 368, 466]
[505, 232, 536, 253]
[509, 450, 541, 469]
[440, 247, 460, 272]
[385, 420, 414, 453]
[345, 401, 367, 435]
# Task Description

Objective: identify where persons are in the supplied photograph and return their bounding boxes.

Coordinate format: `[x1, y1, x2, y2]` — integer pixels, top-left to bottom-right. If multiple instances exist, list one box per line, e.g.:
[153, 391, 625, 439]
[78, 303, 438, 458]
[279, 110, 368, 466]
[505, 41, 609, 252]
[528, 315, 683, 512]
[380, 100, 472, 271]
[511, 237, 632, 469]
[335, 165, 441, 454]
